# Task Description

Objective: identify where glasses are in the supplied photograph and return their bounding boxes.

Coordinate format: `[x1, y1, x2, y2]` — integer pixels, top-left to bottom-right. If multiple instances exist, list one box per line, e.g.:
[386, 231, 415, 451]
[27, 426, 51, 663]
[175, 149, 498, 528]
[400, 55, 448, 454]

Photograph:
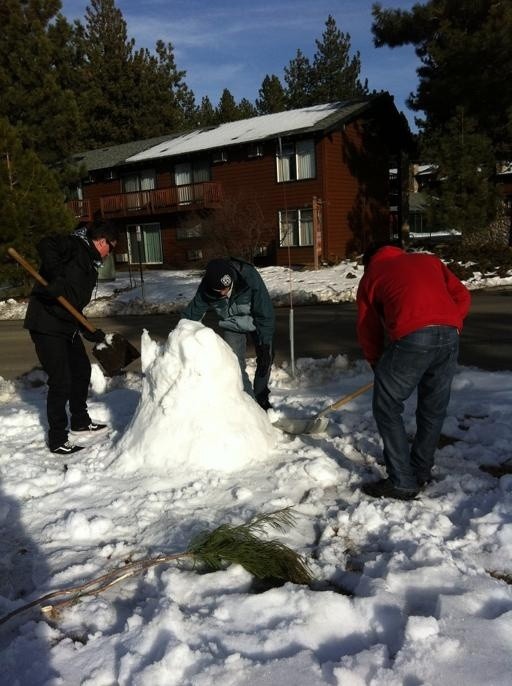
[106, 239, 114, 253]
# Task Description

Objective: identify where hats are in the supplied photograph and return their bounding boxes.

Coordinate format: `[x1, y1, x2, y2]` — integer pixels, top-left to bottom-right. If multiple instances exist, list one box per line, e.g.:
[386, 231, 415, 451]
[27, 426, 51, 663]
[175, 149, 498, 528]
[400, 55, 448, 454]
[205, 260, 235, 289]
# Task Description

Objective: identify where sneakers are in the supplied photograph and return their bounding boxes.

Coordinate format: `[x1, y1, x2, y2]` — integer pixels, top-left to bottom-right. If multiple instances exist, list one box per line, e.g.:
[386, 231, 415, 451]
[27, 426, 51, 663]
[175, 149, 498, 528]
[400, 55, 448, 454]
[50, 443, 84, 454]
[362, 479, 432, 501]
[71, 422, 107, 431]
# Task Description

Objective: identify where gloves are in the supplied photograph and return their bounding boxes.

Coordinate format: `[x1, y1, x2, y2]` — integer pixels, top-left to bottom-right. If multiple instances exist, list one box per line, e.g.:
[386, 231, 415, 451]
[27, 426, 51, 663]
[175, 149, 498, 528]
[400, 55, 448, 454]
[256, 344, 275, 364]
[41, 279, 65, 300]
[83, 329, 106, 343]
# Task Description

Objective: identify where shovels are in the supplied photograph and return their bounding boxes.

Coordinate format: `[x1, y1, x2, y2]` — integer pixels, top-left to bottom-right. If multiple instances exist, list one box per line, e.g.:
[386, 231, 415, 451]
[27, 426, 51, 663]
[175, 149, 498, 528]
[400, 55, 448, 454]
[273, 381, 374, 434]
[8, 247, 141, 377]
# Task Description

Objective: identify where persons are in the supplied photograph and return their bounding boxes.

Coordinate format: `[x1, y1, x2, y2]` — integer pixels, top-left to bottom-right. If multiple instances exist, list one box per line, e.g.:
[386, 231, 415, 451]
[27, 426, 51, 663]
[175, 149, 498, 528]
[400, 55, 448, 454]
[180, 257, 276, 410]
[360, 237, 471, 499]
[23, 223, 120, 453]
